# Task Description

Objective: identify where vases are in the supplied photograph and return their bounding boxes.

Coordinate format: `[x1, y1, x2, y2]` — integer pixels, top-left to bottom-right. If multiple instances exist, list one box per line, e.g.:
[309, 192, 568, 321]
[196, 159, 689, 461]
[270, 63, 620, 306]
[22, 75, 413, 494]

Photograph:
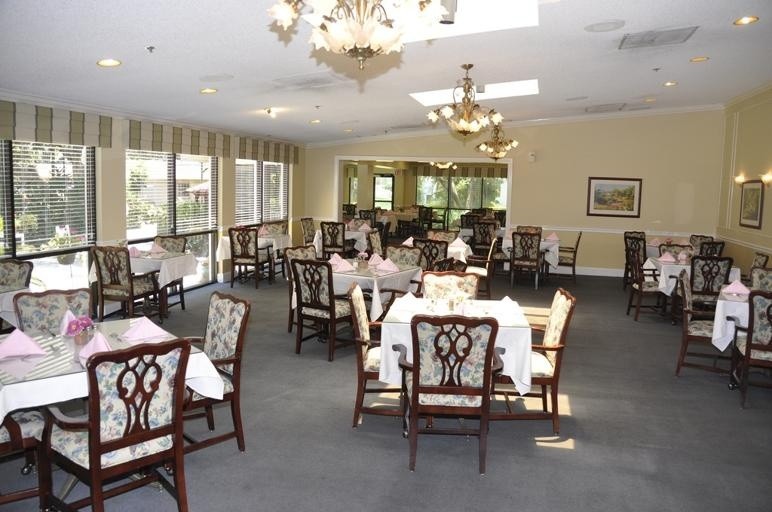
[73, 334, 91, 363]
[358, 259, 368, 270]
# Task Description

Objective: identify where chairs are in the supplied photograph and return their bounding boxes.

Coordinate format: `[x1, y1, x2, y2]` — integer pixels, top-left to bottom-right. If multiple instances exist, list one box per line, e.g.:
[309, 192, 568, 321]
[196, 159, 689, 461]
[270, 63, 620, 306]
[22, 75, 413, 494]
[153, 236, 187, 310]
[420, 271, 480, 301]
[413, 238, 448, 272]
[428, 230, 455, 246]
[402, 207, 423, 238]
[90, 246, 165, 324]
[675, 269, 741, 387]
[742, 252, 769, 286]
[385, 247, 421, 266]
[462, 238, 498, 300]
[730, 290, 772, 409]
[428, 207, 448, 230]
[626, 249, 675, 321]
[510, 231, 544, 290]
[659, 243, 693, 257]
[284, 245, 323, 342]
[376, 222, 383, 238]
[368, 230, 385, 259]
[40, 337, 190, 512]
[343, 204, 355, 221]
[623, 236, 646, 290]
[377, 257, 454, 346]
[472, 209, 486, 216]
[690, 235, 713, 250]
[488, 288, 576, 434]
[348, 282, 431, 429]
[320, 222, 353, 261]
[359, 210, 376, 228]
[381, 222, 391, 260]
[541, 232, 582, 284]
[669, 255, 733, 325]
[461, 215, 479, 229]
[494, 212, 506, 227]
[258, 220, 287, 278]
[164, 291, 252, 474]
[228, 228, 273, 289]
[489, 232, 511, 285]
[700, 241, 725, 257]
[1, 412, 47, 511]
[749, 267, 772, 291]
[472, 222, 495, 256]
[624, 231, 648, 279]
[516, 225, 542, 275]
[301, 218, 315, 246]
[13, 288, 93, 338]
[420, 207, 432, 231]
[0, 259, 33, 334]
[392, 314, 504, 476]
[291, 259, 353, 362]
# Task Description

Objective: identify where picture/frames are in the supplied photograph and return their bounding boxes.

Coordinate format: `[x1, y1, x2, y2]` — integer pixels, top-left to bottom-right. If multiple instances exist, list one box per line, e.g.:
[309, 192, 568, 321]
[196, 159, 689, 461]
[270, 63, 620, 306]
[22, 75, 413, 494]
[586, 177, 642, 218]
[739, 180, 765, 229]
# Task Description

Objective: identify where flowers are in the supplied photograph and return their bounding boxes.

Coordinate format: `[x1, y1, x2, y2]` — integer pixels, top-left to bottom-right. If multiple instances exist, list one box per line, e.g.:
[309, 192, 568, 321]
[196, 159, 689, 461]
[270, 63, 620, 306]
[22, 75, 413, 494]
[66, 315, 95, 344]
[357, 252, 368, 261]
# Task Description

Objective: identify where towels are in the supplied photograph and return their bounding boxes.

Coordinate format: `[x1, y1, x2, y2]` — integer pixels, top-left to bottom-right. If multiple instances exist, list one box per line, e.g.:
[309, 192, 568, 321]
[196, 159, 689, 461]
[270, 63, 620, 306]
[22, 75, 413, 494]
[545, 232, 559, 241]
[333, 260, 356, 273]
[328, 253, 342, 264]
[377, 257, 400, 273]
[493, 296, 524, 316]
[725, 281, 751, 299]
[390, 292, 425, 313]
[656, 250, 677, 265]
[450, 236, 466, 248]
[358, 222, 371, 230]
[402, 237, 414, 248]
[60, 311, 78, 334]
[78, 332, 113, 360]
[152, 244, 168, 253]
[0, 328, 46, 362]
[368, 253, 384, 266]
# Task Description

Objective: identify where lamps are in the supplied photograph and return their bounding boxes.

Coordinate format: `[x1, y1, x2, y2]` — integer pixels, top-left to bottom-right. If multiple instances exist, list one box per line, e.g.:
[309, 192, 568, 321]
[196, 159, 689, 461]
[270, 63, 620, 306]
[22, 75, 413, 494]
[427, 64, 504, 147]
[475, 123, 519, 162]
[267, 0, 433, 70]
